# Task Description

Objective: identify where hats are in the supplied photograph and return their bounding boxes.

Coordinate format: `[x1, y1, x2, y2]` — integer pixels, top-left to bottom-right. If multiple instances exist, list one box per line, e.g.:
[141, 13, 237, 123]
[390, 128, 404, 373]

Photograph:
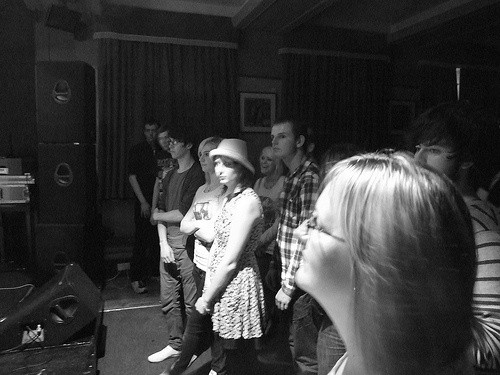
[208, 138, 255, 176]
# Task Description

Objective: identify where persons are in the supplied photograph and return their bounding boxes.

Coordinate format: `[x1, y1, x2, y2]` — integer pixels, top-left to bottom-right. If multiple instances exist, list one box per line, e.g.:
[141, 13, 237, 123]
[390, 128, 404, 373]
[414, 103, 500, 375]
[292, 153, 483, 375]
[128, 119, 366, 374]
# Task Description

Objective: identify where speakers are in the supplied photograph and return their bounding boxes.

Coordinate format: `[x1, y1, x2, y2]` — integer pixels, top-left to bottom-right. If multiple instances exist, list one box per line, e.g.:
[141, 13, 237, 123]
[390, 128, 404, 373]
[32, 61, 98, 142]
[33, 141, 99, 225]
[0, 259, 103, 352]
[37, 221, 96, 284]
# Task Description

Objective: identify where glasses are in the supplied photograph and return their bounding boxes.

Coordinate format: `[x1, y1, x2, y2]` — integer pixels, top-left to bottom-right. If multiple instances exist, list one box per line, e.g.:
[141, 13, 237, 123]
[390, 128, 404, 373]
[167, 139, 192, 145]
[306, 214, 343, 242]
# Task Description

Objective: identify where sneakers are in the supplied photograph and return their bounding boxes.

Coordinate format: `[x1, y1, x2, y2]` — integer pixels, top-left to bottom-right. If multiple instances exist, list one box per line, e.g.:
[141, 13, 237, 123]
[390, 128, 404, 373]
[148, 345, 182, 362]
[131, 280, 148, 294]
